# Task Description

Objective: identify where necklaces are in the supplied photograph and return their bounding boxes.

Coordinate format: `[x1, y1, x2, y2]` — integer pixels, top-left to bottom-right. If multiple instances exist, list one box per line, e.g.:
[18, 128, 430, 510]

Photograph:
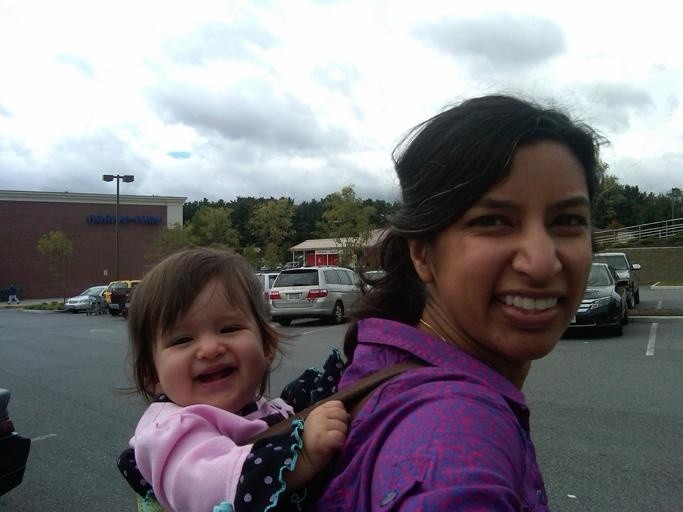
[419, 318, 447, 342]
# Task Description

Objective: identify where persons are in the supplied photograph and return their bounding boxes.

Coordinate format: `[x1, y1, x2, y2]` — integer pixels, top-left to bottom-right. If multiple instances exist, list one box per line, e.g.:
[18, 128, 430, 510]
[7, 282, 19, 305]
[300, 90, 613, 511]
[111, 247, 350, 512]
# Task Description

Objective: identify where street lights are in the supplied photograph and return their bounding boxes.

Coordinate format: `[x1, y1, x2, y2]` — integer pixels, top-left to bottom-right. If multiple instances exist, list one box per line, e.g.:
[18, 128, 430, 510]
[103, 174, 135, 280]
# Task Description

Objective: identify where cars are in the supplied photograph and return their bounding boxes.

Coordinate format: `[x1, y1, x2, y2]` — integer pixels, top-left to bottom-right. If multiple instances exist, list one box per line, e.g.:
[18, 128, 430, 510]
[595, 251, 641, 309]
[255, 272, 282, 318]
[103, 279, 142, 319]
[259, 255, 303, 271]
[64, 285, 106, 313]
[563, 261, 628, 338]
[267, 267, 380, 327]
